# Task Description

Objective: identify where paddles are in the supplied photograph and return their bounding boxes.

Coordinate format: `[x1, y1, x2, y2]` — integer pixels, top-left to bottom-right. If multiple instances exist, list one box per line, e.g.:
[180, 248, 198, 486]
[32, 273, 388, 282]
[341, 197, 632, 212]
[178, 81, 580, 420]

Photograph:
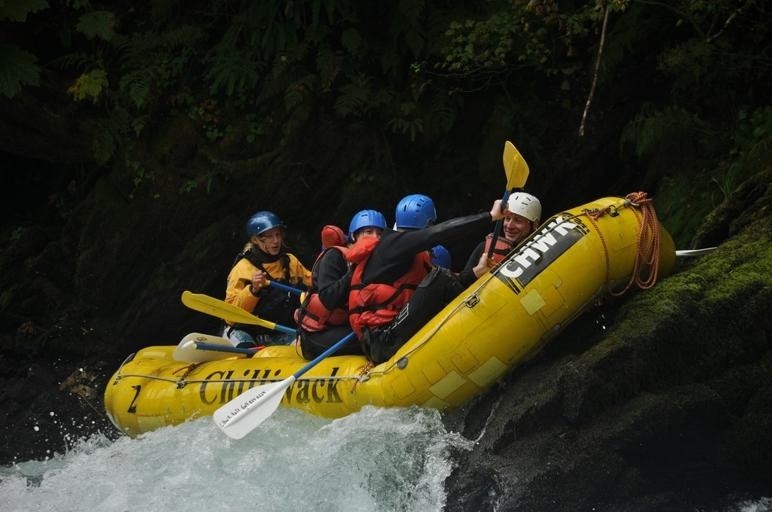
[175, 330, 260, 364]
[213, 333, 354, 439]
[486, 141, 531, 261]
[181, 290, 296, 336]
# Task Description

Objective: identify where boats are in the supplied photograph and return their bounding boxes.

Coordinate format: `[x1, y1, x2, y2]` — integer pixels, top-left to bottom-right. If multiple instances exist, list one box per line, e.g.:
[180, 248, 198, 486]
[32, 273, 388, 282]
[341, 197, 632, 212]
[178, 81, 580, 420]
[104, 198, 676, 438]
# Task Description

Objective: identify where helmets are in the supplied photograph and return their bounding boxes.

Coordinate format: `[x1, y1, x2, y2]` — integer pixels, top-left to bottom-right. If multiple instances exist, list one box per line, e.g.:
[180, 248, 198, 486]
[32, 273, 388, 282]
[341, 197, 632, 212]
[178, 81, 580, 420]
[246, 210, 287, 235]
[430, 245, 452, 268]
[394, 193, 438, 228]
[347, 208, 387, 243]
[502, 191, 543, 222]
